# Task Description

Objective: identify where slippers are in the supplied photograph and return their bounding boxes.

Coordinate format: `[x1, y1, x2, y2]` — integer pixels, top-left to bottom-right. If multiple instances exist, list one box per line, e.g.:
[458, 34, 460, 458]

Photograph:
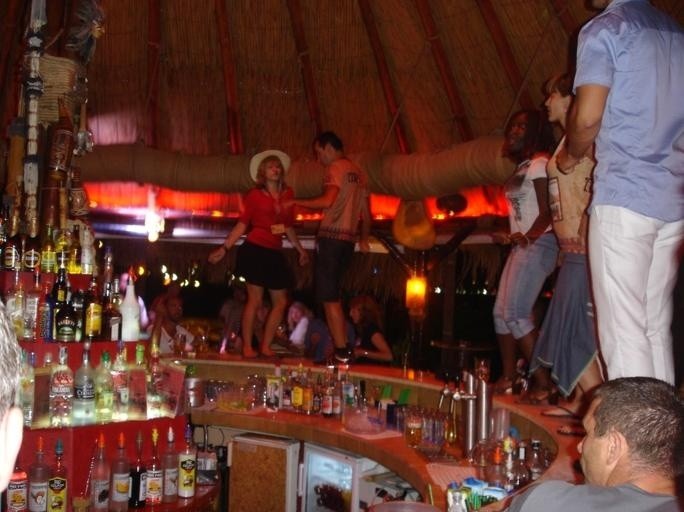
[559, 424, 586, 435]
[541, 407, 583, 419]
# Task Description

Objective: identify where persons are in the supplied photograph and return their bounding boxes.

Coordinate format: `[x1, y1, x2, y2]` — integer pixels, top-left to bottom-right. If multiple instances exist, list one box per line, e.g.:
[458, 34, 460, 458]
[1, 304, 29, 509]
[206, 147, 310, 357]
[527, 67, 611, 435]
[555, 0, 684, 389]
[285, 130, 372, 366]
[117, 272, 395, 366]
[365, 499, 440, 512]
[501, 374, 681, 512]
[485, 108, 561, 405]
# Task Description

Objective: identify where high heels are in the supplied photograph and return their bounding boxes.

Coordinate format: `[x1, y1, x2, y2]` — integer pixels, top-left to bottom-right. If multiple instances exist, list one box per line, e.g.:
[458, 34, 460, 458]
[488, 374, 522, 395]
[515, 383, 559, 404]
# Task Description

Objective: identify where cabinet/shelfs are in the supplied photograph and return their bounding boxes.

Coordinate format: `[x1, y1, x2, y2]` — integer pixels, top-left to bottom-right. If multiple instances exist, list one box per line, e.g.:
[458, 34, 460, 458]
[0, 270, 221, 512]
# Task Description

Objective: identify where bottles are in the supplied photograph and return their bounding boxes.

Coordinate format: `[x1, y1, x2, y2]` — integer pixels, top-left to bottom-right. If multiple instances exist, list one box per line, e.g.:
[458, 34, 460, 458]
[279, 360, 381, 433]
[206, 373, 265, 411]
[183, 364, 203, 406]
[14, 340, 165, 422]
[446, 437, 547, 512]
[7, 263, 140, 340]
[4, 422, 197, 512]
[0, 214, 114, 270]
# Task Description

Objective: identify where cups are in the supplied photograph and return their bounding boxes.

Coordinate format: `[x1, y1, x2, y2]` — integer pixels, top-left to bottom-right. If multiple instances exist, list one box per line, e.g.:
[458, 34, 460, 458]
[174, 334, 185, 354]
[195, 336, 208, 353]
[396, 405, 458, 447]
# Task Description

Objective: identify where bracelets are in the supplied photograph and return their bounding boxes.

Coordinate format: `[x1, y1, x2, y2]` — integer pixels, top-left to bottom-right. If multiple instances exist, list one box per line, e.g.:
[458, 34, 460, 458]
[524, 233, 532, 248]
[220, 243, 229, 252]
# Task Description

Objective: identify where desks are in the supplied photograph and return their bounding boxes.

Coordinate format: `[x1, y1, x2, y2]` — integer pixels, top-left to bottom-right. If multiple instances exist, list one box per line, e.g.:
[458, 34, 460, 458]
[430, 338, 498, 369]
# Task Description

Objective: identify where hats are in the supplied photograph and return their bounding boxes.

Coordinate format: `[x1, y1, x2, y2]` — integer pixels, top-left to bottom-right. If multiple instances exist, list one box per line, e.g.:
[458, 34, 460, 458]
[249, 150, 292, 183]
